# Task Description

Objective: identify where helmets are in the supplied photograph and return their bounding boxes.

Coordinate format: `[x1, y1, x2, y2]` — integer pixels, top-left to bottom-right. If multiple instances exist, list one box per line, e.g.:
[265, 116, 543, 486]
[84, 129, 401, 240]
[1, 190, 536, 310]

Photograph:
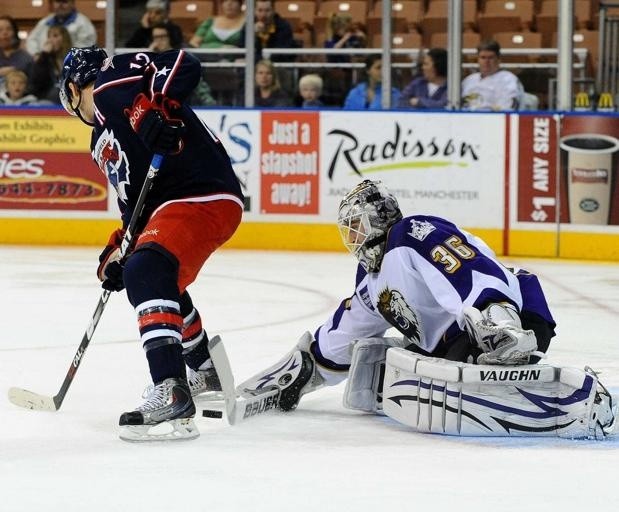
[338, 180, 402, 273]
[59, 46, 113, 117]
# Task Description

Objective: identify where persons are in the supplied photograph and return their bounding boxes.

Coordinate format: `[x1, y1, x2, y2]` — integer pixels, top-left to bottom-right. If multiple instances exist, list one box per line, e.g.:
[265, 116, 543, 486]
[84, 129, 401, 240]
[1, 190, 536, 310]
[124, 1, 186, 49]
[235, 60, 296, 107]
[187, 1, 247, 50]
[225, 178, 615, 443]
[0, 0, 101, 109]
[144, 26, 216, 108]
[341, 54, 402, 110]
[324, 14, 359, 64]
[55, 44, 250, 442]
[240, 1, 295, 60]
[458, 39, 526, 113]
[292, 72, 327, 109]
[400, 46, 461, 111]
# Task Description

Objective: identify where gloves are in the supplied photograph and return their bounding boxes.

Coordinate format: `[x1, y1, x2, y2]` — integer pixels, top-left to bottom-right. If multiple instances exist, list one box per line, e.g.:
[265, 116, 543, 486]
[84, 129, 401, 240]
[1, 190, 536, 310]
[123, 92, 185, 156]
[98, 229, 135, 291]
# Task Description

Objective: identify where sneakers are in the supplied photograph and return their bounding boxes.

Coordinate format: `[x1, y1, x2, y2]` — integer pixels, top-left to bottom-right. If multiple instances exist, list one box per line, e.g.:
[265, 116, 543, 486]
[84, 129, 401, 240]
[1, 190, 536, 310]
[187, 364, 223, 397]
[118, 378, 199, 426]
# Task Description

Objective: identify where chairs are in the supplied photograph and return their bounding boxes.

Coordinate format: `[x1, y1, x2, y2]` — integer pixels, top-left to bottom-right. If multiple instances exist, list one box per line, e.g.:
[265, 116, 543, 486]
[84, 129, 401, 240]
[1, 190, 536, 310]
[0, 0, 617, 107]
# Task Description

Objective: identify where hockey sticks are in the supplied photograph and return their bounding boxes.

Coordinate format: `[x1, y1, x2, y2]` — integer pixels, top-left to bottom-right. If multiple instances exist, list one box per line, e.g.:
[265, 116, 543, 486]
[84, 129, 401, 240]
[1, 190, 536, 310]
[8, 156, 162, 411]
[208, 335, 324, 425]
